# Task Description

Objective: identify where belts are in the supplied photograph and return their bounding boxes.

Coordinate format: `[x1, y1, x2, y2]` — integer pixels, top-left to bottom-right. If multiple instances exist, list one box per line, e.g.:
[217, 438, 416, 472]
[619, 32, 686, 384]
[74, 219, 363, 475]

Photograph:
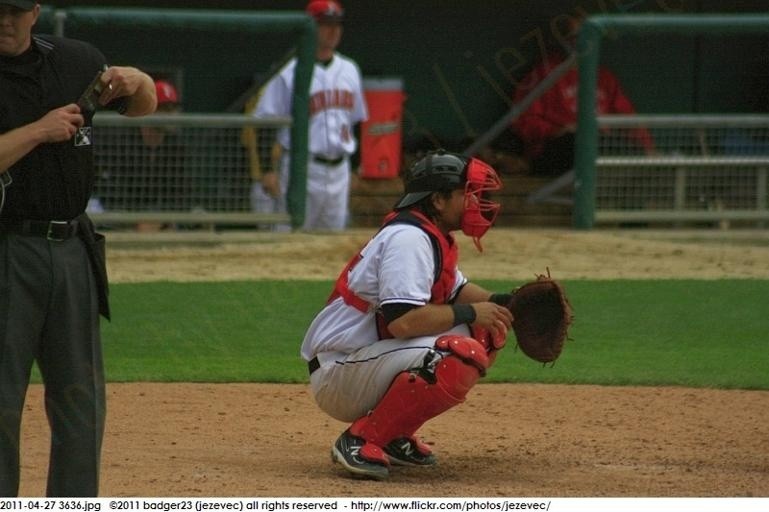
[308, 355, 321, 375]
[314, 153, 347, 167]
[1, 216, 81, 242]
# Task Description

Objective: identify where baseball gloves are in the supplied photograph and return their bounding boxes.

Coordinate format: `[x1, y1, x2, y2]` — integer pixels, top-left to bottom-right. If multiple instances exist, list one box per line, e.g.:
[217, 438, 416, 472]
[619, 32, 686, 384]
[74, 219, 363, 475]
[503, 266, 573, 369]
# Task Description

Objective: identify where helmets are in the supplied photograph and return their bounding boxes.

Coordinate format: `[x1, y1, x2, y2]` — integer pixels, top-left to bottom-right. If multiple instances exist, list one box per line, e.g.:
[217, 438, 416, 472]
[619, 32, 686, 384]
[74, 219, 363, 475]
[392, 148, 472, 212]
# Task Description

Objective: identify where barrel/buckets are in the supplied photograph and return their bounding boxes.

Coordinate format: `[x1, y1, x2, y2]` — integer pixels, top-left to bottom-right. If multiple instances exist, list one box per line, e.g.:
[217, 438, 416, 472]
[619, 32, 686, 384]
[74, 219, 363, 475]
[358, 76, 408, 178]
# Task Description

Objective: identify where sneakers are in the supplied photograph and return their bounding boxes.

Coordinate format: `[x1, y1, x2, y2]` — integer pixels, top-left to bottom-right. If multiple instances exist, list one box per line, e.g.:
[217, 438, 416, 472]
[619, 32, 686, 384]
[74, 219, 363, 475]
[384, 436, 438, 468]
[331, 428, 389, 479]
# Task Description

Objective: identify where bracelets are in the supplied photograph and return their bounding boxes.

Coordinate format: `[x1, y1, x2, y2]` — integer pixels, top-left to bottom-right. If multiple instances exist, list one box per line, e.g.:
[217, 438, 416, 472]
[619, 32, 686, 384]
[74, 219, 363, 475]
[488, 294, 512, 307]
[450, 303, 476, 327]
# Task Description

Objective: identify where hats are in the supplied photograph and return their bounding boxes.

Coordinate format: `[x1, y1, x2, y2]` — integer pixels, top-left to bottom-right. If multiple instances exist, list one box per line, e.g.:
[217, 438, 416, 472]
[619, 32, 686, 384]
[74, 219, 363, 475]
[303, 1, 343, 18]
[153, 81, 179, 105]
[0, 0, 37, 11]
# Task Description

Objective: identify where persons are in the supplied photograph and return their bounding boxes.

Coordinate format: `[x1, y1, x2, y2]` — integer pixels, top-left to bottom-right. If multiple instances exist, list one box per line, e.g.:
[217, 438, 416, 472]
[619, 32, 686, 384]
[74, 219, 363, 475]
[243, 1, 366, 230]
[512, 17, 651, 173]
[300, 148, 572, 481]
[0, 1, 158, 497]
[97, 81, 214, 232]
